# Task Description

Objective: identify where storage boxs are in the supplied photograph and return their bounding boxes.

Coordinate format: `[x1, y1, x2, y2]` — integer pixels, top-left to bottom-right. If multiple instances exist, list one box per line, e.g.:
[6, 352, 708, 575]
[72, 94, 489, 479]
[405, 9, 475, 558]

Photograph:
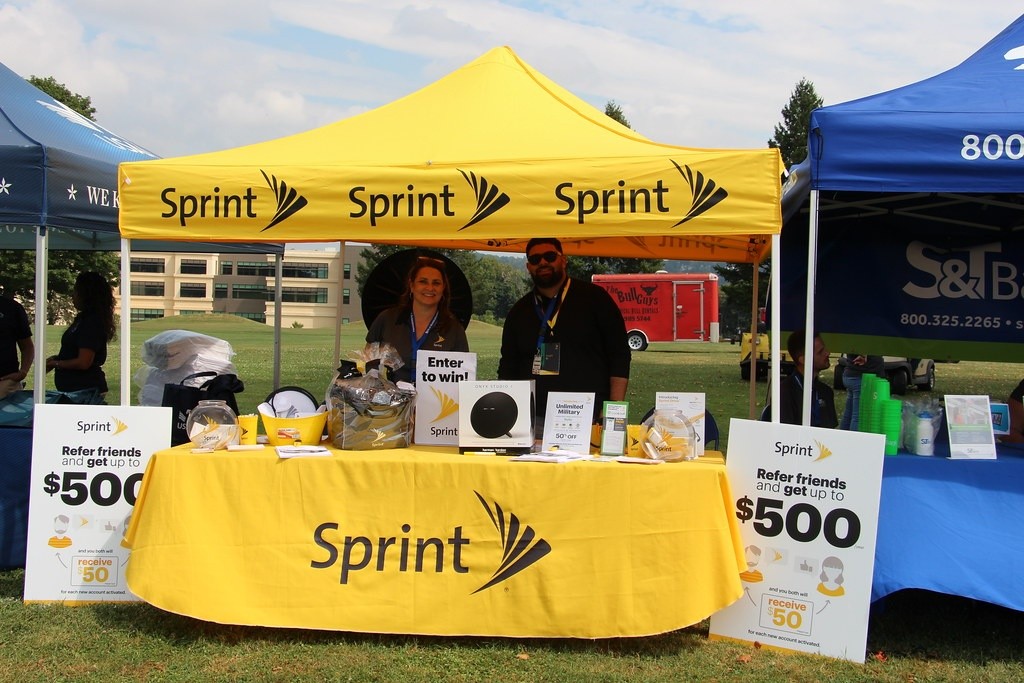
[458, 379, 537, 447]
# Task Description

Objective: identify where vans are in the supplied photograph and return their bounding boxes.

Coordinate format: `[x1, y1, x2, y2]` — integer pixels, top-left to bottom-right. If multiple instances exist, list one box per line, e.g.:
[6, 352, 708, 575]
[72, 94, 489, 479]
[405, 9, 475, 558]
[594, 276, 722, 350]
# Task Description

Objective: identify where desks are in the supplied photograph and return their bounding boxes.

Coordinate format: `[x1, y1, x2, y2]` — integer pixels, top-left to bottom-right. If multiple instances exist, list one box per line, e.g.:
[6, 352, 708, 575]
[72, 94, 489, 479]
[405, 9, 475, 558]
[0, 426, 32, 569]
[871, 443, 1024, 612]
[122, 435, 750, 641]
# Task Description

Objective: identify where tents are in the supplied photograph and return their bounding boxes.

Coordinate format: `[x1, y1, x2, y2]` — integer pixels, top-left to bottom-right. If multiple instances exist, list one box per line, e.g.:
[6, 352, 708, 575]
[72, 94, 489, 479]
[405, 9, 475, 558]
[0, 61, 285, 407]
[784, 11, 1024, 426]
[117, 44, 784, 427]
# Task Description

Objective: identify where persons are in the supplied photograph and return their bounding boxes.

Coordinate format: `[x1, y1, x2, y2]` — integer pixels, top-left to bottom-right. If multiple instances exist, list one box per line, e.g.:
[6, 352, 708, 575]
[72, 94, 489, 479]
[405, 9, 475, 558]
[0, 296, 34, 400]
[778, 329, 839, 429]
[365, 260, 469, 384]
[839, 353, 885, 431]
[46, 270, 116, 399]
[998, 379, 1024, 442]
[496, 238, 632, 437]
[952, 406, 964, 425]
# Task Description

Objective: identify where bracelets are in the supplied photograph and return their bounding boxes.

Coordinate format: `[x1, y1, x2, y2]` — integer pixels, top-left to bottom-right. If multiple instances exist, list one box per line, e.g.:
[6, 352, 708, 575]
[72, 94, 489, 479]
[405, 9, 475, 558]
[56, 361, 60, 370]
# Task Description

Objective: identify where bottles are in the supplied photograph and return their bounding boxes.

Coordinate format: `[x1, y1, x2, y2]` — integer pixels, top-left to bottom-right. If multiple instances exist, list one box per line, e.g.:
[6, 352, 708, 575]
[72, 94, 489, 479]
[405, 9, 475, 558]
[915, 410, 935, 457]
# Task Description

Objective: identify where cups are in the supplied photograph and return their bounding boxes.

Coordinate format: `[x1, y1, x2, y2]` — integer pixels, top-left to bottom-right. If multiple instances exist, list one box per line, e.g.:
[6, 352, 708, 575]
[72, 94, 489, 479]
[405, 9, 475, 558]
[663, 436, 689, 463]
[857, 373, 903, 455]
[238, 414, 258, 446]
[626, 424, 649, 458]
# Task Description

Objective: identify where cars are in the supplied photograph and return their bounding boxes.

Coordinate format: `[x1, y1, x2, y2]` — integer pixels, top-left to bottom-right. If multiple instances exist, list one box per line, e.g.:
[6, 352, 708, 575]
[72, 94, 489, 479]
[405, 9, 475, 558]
[835, 353, 938, 390]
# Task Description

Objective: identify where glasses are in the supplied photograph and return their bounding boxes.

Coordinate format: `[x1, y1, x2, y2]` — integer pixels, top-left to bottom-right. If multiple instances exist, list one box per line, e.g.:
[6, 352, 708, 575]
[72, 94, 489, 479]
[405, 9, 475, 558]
[417, 256, 446, 266]
[527, 250, 563, 264]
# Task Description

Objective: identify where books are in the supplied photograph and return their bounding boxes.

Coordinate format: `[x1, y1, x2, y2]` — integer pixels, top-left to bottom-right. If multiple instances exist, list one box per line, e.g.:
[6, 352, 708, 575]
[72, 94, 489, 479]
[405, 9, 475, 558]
[275, 445, 332, 459]
[512, 452, 594, 462]
[227, 444, 264, 451]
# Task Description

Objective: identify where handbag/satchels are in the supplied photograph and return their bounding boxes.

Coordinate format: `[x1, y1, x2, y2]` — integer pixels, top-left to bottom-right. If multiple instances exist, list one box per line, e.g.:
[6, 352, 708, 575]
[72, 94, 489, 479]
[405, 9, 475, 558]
[833, 364, 847, 392]
[161, 371, 244, 448]
